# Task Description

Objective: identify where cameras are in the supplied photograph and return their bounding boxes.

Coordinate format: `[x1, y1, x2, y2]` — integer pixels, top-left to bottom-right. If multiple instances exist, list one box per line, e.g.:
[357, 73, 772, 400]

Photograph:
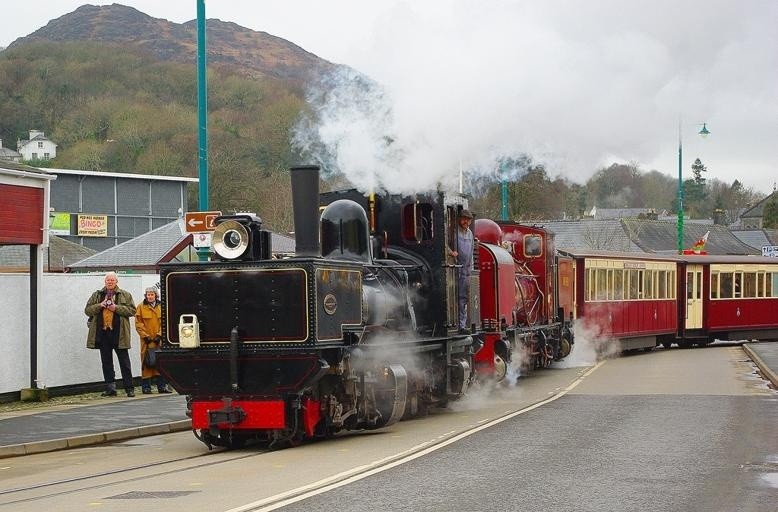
[105, 304, 112, 308]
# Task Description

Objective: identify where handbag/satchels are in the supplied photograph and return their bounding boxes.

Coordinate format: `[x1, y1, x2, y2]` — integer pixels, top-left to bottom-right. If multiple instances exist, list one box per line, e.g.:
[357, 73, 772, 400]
[144, 339, 163, 367]
[87, 316, 94, 328]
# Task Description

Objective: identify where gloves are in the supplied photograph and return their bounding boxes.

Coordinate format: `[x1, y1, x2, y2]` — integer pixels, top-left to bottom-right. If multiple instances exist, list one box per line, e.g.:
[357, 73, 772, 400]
[153, 336, 161, 344]
[144, 337, 151, 344]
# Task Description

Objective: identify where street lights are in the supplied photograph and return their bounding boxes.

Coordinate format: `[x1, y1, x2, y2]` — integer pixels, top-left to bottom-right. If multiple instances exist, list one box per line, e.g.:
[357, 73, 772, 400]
[674, 120, 712, 253]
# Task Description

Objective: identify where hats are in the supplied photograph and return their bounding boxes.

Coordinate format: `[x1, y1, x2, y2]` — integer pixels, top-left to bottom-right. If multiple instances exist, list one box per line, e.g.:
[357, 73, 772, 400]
[458, 209, 474, 219]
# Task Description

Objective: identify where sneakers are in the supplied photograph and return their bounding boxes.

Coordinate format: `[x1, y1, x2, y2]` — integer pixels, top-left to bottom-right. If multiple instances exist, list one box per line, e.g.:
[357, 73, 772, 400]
[127, 392, 136, 397]
[157, 388, 172, 393]
[101, 390, 117, 397]
[142, 388, 153, 394]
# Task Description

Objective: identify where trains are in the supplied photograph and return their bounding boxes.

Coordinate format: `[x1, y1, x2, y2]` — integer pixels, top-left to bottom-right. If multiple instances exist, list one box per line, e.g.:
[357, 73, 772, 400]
[145, 156, 776, 446]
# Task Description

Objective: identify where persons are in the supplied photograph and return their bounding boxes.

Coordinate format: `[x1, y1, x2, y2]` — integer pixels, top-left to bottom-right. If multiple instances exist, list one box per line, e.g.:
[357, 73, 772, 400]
[720, 274, 740, 297]
[134, 286, 173, 394]
[84, 270, 137, 397]
[447, 208, 476, 335]
